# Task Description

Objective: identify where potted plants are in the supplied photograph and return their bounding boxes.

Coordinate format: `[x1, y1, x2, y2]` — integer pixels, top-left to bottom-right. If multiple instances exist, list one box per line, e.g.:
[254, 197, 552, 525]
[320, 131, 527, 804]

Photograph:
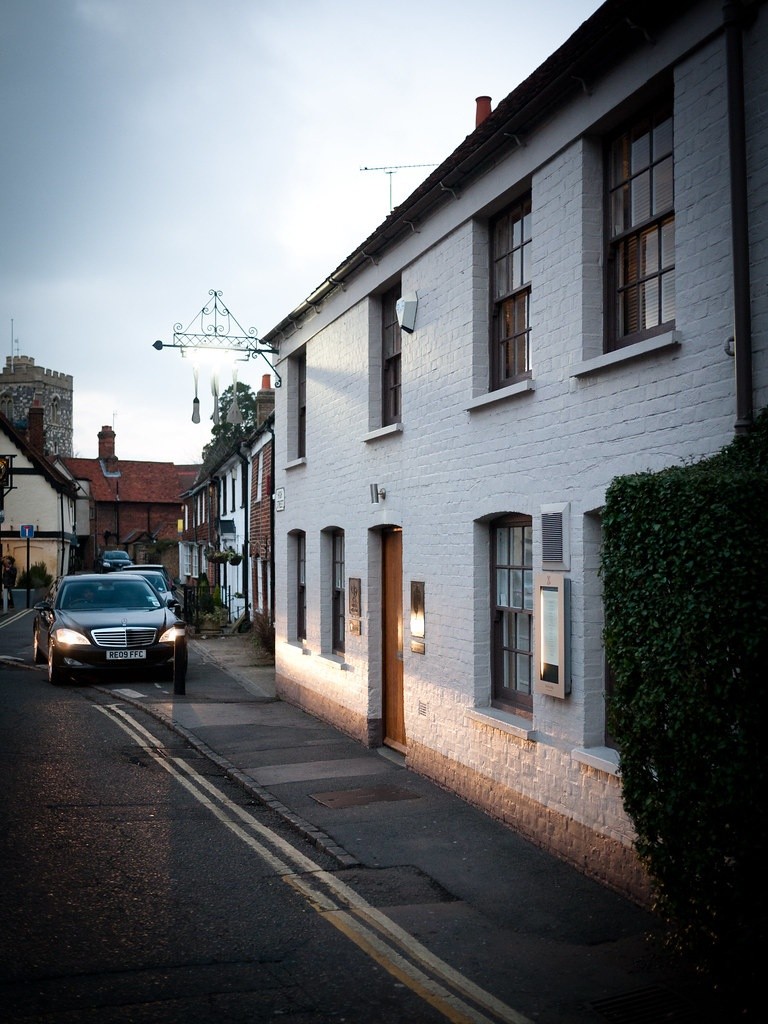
[213, 606, 228, 626]
[11, 561, 54, 610]
[226, 548, 243, 566]
[214, 552, 228, 564]
[199, 612, 220, 630]
[204, 546, 214, 562]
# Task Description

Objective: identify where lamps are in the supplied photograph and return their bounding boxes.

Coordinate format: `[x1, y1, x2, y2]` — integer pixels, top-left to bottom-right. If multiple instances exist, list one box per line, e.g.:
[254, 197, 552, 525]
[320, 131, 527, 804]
[395, 290, 418, 334]
[152, 289, 281, 425]
[370, 483, 386, 504]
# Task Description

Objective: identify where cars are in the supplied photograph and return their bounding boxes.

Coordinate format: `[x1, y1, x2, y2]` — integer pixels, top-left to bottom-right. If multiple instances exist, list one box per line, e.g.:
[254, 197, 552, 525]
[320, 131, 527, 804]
[31, 573, 188, 688]
[112, 570, 182, 624]
[121, 564, 173, 588]
[93, 550, 131, 577]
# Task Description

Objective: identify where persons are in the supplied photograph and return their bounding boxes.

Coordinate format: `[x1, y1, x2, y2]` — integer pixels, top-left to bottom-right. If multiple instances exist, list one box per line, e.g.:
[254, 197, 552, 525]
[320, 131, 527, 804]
[82, 587, 98, 603]
[2, 556, 17, 610]
